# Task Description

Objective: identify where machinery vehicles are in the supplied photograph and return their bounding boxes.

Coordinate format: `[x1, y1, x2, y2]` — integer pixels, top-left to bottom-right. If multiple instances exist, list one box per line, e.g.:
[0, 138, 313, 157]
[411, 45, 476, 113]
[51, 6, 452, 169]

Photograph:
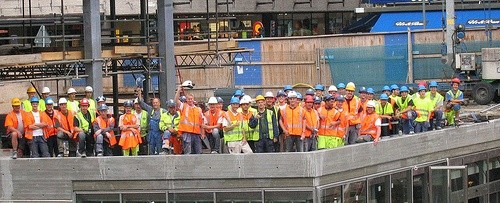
[413, 23, 500, 105]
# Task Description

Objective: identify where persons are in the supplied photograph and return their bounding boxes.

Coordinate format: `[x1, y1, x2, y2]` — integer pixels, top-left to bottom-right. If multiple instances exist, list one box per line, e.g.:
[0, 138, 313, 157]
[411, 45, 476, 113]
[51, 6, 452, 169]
[248, 95, 278, 153]
[279, 90, 307, 152]
[137, 86, 168, 155]
[337, 82, 345, 93]
[4, 85, 148, 158]
[195, 88, 254, 153]
[305, 89, 315, 96]
[327, 84, 337, 93]
[314, 84, 323, 95]
[263, 91, 278, 131]
[175, 85, 204, 154]
[342, 82, 362, 145]
[276, 89, 287, 109]
[296, 91, 302, 100]
[284, 85, 292, 95]
[316, 93, 342, 150]
[159, 100, 179, 153]
[313, 96, 322, 114]
[334, 96, 347, 145]
[357, 78, 470, 146]
[301, 95, 319, 151]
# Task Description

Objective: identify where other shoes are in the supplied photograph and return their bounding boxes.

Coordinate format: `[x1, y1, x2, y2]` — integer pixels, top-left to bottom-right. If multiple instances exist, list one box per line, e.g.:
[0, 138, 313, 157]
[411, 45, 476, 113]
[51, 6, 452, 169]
[58, 153, 63, 157]
[81, 152, 86, 157]
[159, 152, 168, 155]
[435, 124, 441, 130]
[13, 151, 17, 159]
[211, 150, 218, 154]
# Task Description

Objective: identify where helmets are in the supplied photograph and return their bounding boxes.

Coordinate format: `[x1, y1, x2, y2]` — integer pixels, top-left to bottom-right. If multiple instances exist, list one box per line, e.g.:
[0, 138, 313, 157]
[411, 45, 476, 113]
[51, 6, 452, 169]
[27, 87, 36, 93]
[181, 80, 194, 90]
[59, 98, 67, 105]
[80, 98, 89, 106]
[166, 99, 176, 107]
[31, 97, 39, 103]
[208, 82, 437, 109]
[11, 99, 20, 106]
[99, 105, 108, 111]
[67, 88, 76, 94]
[96, 96, 106, 102]
[136, 76, 146, 89]
[85, 86, 93, 93]
[42, 87, 51, 93]
[45, 98, 53, 105]
[134, 98, 141, 104]
[124, 102, 133, 108]
[452, 78, 460, 85]
